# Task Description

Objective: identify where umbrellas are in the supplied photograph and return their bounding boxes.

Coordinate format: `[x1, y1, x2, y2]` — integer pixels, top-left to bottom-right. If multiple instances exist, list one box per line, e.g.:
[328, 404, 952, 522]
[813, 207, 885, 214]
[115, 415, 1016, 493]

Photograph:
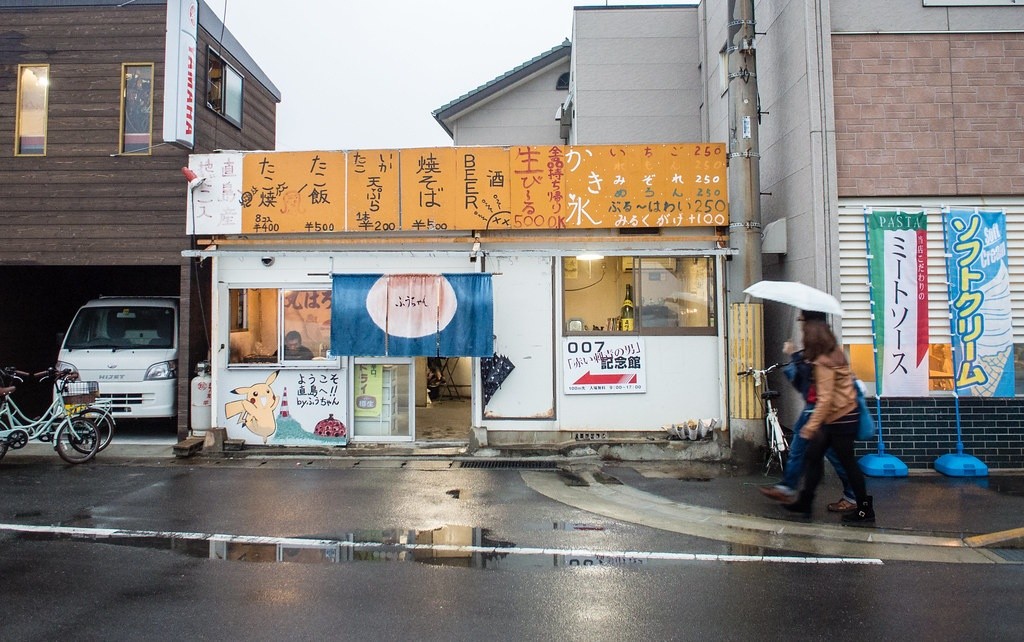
[481, 336, 514, 405]
[744, 280, 844, 342]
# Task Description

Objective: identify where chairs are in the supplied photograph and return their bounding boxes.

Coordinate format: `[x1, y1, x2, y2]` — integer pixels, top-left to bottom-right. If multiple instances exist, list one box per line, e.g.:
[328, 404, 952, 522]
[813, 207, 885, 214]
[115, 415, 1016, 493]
[104, 318, 133, 348]
[149, 320, 174, 348]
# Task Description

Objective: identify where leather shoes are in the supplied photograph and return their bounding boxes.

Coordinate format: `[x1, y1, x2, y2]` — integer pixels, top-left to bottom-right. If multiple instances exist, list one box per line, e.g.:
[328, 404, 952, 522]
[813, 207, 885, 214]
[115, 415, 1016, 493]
[827, 497, 858, 511]
[759, 485, 800, 502]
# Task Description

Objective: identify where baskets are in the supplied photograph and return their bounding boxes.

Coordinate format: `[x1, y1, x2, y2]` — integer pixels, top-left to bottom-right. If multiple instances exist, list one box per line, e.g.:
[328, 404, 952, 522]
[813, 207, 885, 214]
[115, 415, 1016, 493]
[61, 381, 99, 405]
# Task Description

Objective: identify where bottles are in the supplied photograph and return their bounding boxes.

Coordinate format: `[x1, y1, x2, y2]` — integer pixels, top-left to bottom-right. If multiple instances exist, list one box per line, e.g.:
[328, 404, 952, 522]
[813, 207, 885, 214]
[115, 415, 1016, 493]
[607, 318, 621, 331]
[593, 325, 603, 331]
[319, 343, 330, 358]
[621, 284, 634, 331]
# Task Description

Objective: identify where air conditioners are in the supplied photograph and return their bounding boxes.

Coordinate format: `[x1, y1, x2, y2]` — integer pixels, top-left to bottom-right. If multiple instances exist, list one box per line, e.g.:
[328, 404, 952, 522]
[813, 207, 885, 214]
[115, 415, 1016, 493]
[621, 256, 676, 274]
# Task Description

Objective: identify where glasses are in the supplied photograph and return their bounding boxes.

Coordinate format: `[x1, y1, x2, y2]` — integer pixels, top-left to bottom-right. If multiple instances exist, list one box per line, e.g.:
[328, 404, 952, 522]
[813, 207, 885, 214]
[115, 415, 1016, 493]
[796, 317, 806, 322]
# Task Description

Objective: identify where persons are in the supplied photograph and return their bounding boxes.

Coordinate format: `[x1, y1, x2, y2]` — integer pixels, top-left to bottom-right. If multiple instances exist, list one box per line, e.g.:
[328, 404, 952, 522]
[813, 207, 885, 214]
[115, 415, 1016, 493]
[275, 331, 312, 357]
[757, 309, 875, 522]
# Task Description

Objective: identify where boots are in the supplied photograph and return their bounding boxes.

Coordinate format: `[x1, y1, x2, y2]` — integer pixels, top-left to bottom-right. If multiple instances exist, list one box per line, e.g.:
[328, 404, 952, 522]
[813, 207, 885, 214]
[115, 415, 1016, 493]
[777, 497, 814, 519]
[841, 496, 875, 524]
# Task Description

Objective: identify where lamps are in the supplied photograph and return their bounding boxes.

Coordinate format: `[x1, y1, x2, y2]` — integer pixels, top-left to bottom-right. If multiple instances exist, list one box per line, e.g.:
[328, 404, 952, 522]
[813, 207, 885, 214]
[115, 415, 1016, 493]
[181, 167, 206, 193]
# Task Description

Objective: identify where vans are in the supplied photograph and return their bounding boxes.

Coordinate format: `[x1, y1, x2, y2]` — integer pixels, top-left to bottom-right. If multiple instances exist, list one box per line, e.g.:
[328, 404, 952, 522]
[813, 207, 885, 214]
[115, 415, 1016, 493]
[51, 296, 181, 420]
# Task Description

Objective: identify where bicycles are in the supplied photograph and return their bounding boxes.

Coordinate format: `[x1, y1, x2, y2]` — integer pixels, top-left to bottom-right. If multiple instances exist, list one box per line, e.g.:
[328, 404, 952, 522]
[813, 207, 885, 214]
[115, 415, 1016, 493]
[0, 363, 116, 466]
[734, 362, 788, 478]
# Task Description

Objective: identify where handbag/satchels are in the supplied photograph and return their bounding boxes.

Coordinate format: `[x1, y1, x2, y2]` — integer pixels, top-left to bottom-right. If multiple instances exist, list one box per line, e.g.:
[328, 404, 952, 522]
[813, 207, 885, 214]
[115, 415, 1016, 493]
[854, 379, 876, 441]
